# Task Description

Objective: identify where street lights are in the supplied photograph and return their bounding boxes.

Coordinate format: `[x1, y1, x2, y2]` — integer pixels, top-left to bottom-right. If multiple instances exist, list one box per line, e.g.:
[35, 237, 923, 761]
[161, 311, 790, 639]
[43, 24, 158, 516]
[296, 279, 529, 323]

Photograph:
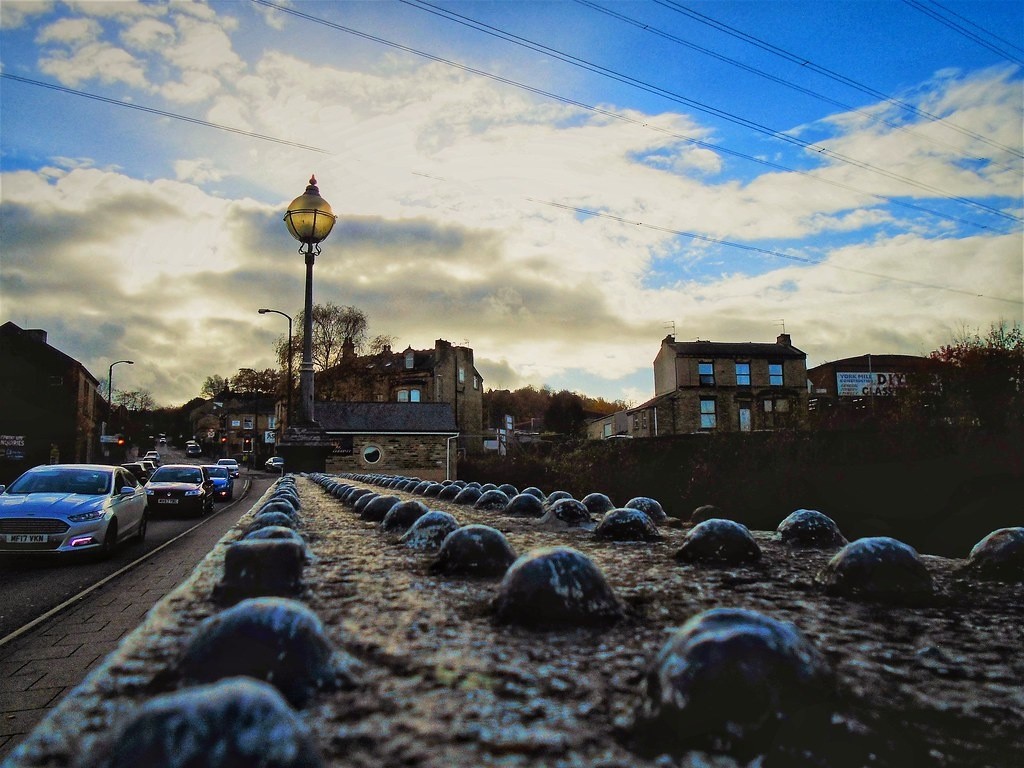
[282, 173, 337, 426]
[238, 367, 259, 470]
[105, 360, 135, 435]
[258, 308, 293, 423]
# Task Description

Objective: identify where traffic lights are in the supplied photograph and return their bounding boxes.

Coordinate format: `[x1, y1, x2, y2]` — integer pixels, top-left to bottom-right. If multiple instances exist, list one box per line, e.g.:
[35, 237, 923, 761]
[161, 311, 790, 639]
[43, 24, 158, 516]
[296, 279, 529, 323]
[243, 435, 251, 451]
[220, 434, 226, 444]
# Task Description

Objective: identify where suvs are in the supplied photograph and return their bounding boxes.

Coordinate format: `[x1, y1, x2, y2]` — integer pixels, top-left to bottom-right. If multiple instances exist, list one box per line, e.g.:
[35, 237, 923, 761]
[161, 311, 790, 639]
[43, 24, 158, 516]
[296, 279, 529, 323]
[185, 445, 202, 458]
[119, 460, 163, 486]
[144, 451, 161, 462]
[159, 438, 166, 444]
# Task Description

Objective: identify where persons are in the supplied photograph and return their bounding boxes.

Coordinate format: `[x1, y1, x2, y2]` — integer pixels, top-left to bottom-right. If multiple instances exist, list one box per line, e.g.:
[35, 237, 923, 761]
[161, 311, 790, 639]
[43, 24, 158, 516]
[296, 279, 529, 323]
[50, 441, 60, 464]
[242, 453, 248, 468]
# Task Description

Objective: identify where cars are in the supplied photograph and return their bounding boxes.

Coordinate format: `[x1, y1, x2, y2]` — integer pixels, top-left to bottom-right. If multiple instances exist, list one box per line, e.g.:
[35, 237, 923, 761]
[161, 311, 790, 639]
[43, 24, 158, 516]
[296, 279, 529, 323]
[0, 464, 150, 562]
[264, 457, 285, 473]
[216, 459, 240, 479]
[199, 464, 234, 503]
[143, 464, 215, 519]
